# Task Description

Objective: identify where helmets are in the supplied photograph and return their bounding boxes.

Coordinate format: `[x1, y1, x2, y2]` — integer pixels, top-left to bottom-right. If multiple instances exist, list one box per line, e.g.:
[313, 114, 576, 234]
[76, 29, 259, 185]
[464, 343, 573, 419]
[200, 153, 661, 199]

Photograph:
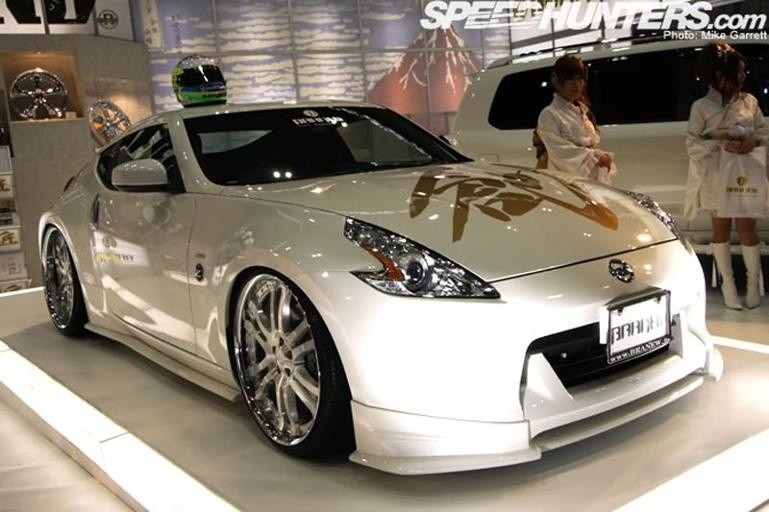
[171, 55, 227, 108]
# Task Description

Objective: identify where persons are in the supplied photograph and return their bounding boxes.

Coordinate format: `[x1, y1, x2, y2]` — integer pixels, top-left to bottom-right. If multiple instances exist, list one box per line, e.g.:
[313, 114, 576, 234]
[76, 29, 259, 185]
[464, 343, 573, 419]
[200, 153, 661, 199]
[532, 51, 615, 183]
[683, 39, 768, 311]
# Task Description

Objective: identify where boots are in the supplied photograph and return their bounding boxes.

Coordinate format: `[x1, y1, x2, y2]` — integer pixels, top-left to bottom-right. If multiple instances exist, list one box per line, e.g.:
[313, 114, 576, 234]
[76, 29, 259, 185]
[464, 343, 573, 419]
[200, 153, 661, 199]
[711, 240, 742, 310]
[741, 242, 762, 310]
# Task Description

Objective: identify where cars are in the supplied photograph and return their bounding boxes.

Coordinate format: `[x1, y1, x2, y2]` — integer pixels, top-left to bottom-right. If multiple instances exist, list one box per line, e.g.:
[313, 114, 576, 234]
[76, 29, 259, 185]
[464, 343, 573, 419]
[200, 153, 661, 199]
[37, 101, 723, 475]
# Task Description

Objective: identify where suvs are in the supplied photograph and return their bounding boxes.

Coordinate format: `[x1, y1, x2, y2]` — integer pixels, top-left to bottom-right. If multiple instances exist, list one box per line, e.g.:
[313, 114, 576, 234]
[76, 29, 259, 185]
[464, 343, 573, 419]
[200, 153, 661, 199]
[444, 33, 768, 262]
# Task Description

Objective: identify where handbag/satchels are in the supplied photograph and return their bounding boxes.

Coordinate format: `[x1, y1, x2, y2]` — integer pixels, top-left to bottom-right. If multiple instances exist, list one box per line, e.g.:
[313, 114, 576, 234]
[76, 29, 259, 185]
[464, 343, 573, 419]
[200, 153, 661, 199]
[712, 143, 767, 220]
